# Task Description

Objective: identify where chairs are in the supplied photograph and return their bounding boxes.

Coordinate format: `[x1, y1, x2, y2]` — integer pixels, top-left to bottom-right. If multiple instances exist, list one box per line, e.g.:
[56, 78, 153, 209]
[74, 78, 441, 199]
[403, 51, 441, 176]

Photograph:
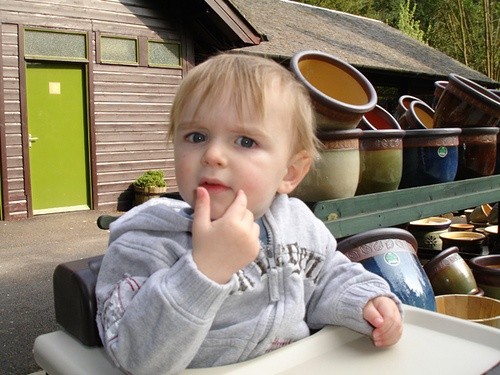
[53, 254, 107, 347]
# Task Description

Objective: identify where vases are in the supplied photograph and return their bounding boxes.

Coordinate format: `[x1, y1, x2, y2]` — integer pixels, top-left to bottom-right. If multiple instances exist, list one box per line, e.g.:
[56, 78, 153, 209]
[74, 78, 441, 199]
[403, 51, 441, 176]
[290, 49, 500, 330]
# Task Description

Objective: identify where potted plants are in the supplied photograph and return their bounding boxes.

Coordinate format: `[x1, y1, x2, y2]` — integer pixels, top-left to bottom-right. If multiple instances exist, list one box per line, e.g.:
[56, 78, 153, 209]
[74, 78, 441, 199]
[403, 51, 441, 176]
[133, 170, 167, 206]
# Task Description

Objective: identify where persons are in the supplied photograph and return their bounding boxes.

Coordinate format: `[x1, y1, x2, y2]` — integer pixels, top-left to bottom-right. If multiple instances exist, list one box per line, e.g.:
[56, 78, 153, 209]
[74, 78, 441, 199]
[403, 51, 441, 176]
[93, 52, 406, 375]
